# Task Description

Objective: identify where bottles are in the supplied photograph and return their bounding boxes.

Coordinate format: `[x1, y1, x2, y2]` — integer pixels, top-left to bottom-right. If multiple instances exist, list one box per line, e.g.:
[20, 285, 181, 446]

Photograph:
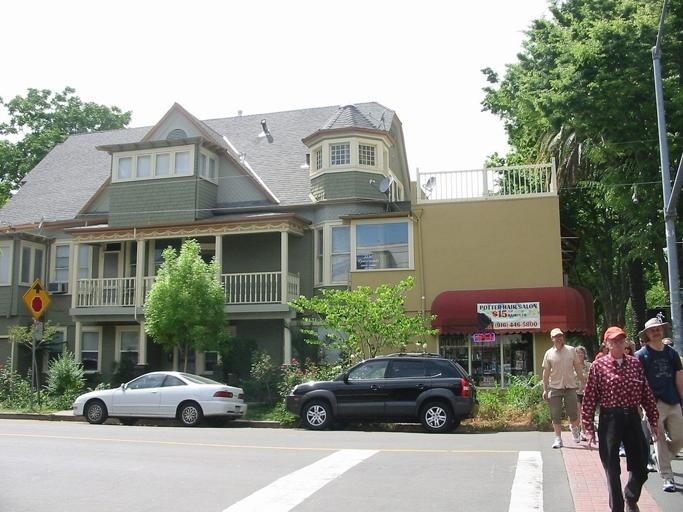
[549, 328, 564, 338]
[638, 316, 670, 341]
[604, 326, 628, 341]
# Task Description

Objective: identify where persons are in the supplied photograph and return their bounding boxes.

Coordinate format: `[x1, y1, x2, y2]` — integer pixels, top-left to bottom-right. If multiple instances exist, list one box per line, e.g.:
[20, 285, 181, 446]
[631, 317, 683, 492]
[571, 333, 682, 481]
[539, 327, 588, 447]
[578, 326, 663, 511]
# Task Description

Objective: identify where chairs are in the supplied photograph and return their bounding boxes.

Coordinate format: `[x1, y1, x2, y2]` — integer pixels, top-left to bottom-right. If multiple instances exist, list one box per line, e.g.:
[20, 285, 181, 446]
[619, 446, 626, 457]
[552, 438, 563, 448]
[662, 478, 676, 492]
[626, 499, 638, 512]
[570, 424, 587, 443]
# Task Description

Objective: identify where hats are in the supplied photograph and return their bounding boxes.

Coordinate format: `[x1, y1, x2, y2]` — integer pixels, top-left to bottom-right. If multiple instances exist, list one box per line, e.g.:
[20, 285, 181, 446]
[72, 369, 248, 425]
[285, 353, 478, 433]
